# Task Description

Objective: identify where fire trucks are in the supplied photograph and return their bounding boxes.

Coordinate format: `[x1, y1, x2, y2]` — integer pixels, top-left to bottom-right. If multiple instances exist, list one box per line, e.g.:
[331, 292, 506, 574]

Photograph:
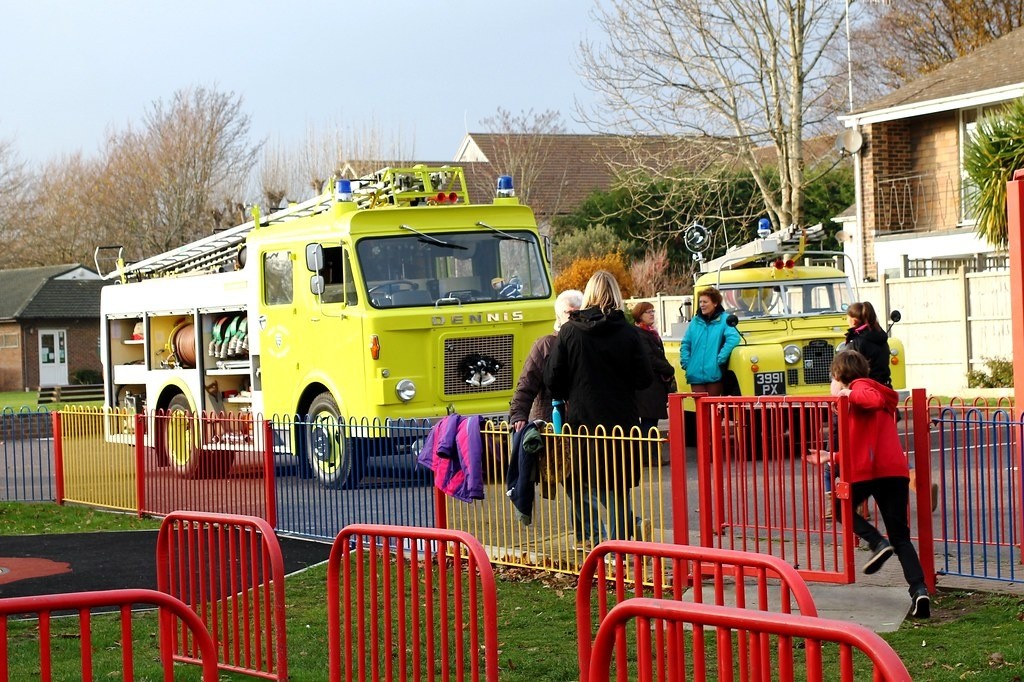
[97, 163, 566, 492]
[610, 218, 912, 453]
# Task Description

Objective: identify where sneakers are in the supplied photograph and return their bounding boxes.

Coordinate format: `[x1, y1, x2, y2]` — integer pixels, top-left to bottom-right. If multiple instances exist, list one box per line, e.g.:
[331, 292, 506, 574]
[862, 539, 893, 574]
[910, 588, 930, 619]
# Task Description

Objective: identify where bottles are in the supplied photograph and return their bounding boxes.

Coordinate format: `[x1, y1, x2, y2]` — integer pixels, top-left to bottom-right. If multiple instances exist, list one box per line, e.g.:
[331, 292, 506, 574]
[552, 399, 566, 434]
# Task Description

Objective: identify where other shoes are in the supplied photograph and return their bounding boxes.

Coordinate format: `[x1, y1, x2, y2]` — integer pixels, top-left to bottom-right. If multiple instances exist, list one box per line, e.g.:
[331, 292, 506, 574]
[636, 519, 651, 565]
[572, 538, 608, 552]
[931, 484, 938, 512]
[604, 554, 626, 566]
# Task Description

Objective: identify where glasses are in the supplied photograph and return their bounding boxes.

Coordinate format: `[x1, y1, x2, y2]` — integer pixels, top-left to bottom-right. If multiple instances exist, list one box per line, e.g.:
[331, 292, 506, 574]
[643, 310, 655, 315]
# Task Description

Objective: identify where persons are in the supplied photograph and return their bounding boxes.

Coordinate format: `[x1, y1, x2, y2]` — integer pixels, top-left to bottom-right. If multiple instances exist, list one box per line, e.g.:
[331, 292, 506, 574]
[631, 302, 656, 330]
[541, 270, 678, 543]
[823, 304, 939, 520]
[510, 270, 606, 552]
[808, 353, 932, 621]
[681, 288, 743, 464]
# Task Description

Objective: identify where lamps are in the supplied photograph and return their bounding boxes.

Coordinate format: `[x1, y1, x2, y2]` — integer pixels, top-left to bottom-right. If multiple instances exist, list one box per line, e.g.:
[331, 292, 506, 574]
[840, 147, 852, 156]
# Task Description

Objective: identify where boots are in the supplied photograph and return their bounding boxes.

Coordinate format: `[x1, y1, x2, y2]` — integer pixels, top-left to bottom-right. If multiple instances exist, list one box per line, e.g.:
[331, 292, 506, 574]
[824, 491, 837, 521]
[857, 503, 871, 521]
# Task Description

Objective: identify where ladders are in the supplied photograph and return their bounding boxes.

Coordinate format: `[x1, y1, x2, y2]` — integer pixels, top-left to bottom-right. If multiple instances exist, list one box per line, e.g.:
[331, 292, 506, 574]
[698, 222, 831, 275]
[93, 167, 453, 280]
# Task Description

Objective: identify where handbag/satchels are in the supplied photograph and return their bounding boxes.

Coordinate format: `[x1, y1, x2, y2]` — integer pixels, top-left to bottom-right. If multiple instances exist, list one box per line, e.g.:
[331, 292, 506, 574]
[885, 383, 901, 423]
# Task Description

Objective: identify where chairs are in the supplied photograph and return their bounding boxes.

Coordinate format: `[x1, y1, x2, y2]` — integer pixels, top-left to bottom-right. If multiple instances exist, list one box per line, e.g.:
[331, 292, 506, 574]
[439, 276, 481, 299]
